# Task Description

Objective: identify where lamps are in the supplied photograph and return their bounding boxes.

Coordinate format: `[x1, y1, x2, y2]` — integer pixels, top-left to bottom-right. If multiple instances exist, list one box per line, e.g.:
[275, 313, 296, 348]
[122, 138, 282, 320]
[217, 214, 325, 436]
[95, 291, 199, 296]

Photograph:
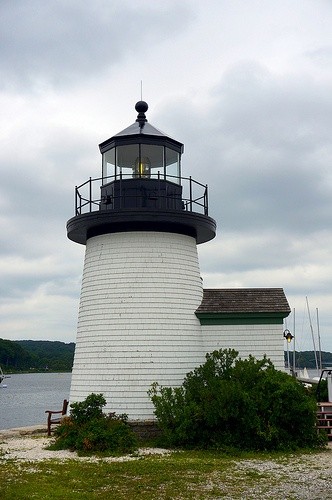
[283, 329, 294, 343]
